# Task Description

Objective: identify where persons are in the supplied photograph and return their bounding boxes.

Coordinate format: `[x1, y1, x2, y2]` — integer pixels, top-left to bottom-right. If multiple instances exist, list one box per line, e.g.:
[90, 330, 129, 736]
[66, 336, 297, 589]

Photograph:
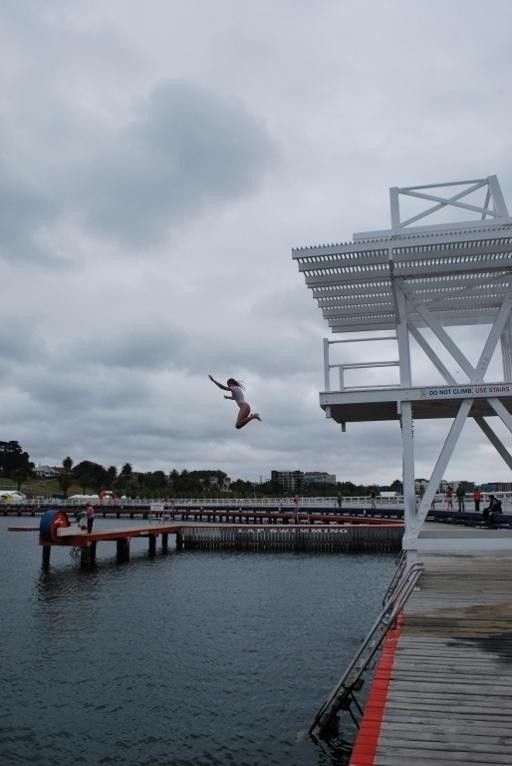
[337, 492, 343, 507]
[294, 494, 298, 506]
[86, 503, 96, 534]
[473, 488, 480, 512]
[368, 489, 376, 509]
[208, 374, 262, 430]
[481, 495, 494, 522]
[487, 498, 502, 520]
[430, 497, 435, 509]
[444, 486, 453, 511]
[455, 484, 465, 513]
[419, 485, 424, 500]
